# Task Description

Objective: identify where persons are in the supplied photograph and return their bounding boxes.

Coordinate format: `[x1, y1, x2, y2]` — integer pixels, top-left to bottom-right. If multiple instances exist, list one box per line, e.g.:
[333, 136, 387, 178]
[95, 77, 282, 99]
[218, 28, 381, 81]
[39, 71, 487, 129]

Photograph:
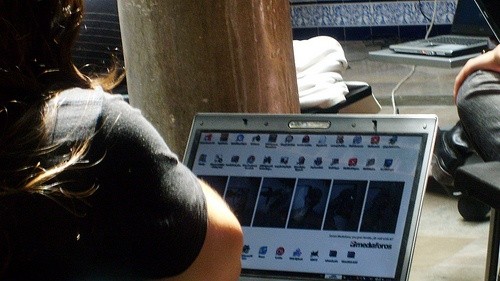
[0, 0, 242, 281]
[430, 44, 500, 223]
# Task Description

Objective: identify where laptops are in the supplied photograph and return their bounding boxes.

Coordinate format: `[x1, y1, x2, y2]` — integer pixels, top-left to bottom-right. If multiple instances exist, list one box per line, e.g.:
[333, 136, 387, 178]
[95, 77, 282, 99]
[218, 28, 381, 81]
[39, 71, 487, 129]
[181, 113, 439, 281]
[389, 0, 500, 57]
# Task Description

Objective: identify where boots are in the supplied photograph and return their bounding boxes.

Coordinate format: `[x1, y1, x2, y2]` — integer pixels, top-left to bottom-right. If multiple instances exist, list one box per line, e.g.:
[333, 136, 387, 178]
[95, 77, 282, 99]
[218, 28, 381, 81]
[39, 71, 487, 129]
[431, 121, 472, 183]
